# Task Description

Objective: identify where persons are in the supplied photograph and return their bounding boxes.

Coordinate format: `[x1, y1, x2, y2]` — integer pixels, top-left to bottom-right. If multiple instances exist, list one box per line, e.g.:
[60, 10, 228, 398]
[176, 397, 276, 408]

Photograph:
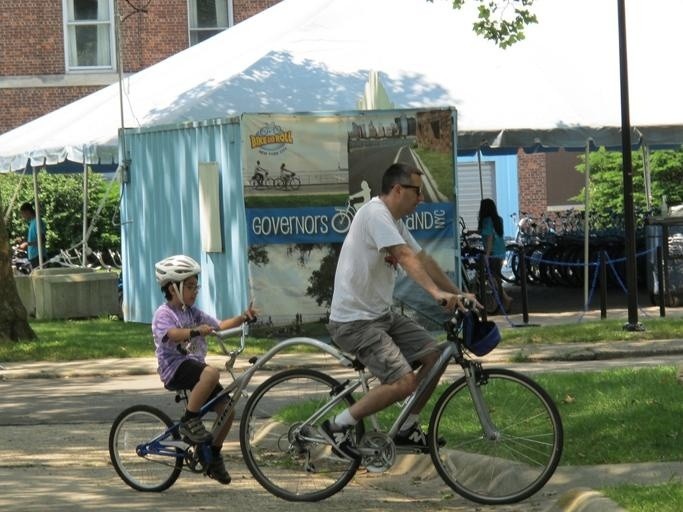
[254, 160, 264, 186]
[280, 163, 295, 189]
[14, 203, 46, 269]
[151, 254, 256, 484]
[316, 164, 486, 462]
[478, 198, 515, 314]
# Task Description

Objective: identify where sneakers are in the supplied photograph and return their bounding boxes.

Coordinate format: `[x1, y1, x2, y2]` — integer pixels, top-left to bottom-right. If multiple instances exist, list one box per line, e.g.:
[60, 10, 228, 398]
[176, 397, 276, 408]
[505, 296, 514, 314]
[198, 445, 231, 484]
[318, 414, 362, 460]
[179, 416, 212, 443]
[393, 420, 446, 448]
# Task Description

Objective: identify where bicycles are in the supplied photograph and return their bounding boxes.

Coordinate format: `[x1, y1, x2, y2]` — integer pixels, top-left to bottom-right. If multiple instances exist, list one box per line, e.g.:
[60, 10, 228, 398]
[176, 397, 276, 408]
[273, 175, 301, 191]
[249, 174, 273, 190]
[461, 207, 644, 315]
[331, 198, 357, 234]
[108, 297, 563, 505]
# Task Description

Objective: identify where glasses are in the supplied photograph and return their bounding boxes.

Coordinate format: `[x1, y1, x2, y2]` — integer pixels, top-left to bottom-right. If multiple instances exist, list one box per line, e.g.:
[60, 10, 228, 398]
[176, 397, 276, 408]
[392, 184, 421, 194]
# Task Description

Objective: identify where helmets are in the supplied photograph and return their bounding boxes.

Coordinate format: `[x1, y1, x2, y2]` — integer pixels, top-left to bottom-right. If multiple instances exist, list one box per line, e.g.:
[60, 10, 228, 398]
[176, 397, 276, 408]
[153, 255, 201, 289]
